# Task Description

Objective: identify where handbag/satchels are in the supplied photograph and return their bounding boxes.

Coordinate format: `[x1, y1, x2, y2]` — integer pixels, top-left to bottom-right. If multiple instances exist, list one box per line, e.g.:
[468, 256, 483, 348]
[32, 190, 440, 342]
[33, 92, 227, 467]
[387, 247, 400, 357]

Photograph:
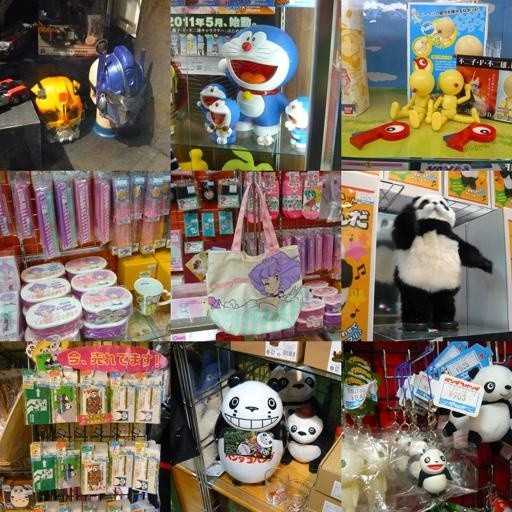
[200, 181, 306, 338]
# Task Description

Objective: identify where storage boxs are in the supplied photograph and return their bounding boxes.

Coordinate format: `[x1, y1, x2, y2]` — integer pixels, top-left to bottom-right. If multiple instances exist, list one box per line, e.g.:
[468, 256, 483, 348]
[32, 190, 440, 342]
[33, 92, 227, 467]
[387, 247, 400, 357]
[303, 432, 390, 512]
[226, 338, 345, 379]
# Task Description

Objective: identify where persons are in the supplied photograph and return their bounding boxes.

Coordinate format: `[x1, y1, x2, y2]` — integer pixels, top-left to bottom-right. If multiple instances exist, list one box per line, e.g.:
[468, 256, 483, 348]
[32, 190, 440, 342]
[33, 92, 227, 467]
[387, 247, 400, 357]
[390, 197, 493, 330]
